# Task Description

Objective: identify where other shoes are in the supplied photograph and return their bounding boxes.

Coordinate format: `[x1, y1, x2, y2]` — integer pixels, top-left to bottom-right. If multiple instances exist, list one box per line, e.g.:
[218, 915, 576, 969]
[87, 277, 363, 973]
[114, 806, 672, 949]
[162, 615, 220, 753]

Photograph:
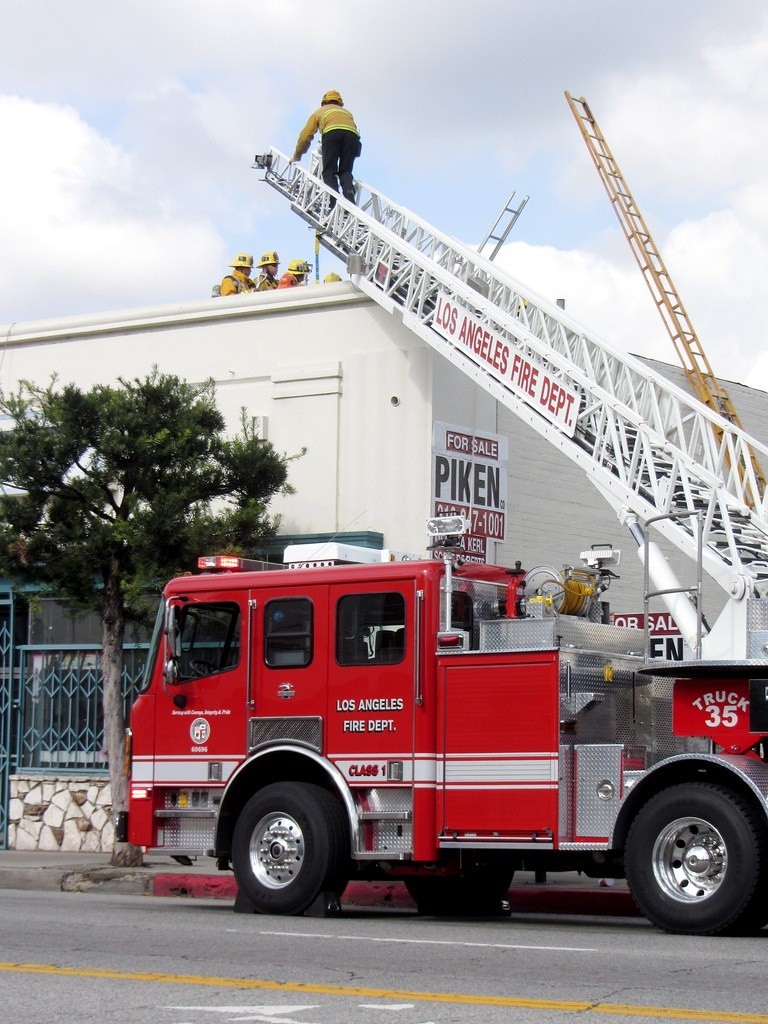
[345, 189, 356, 205]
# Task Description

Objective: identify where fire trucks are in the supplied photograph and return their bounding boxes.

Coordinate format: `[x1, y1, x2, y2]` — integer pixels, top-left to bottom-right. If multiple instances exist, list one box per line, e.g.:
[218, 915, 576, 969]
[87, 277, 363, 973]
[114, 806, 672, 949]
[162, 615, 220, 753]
[110, 145, 768, 939]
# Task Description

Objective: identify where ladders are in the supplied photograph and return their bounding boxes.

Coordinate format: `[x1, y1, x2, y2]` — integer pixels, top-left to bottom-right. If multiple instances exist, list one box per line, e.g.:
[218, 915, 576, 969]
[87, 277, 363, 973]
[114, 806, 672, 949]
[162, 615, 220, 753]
[478, 191, 529, 262]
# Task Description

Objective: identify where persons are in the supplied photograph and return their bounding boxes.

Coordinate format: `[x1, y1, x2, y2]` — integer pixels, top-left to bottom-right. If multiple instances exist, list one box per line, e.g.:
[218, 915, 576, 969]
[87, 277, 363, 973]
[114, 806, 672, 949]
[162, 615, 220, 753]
[220, 251, 343, 296]
[288, 90, 363, 215]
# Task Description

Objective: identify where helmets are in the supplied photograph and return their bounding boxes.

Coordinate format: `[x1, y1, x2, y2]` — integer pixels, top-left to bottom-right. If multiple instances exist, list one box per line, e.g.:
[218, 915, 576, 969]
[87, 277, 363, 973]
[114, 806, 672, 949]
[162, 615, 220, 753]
[228, 253, 254, 268]
[323, 273, 342, 283]
[286, 259, 311, 275]
[321, 90, 344, 107]
[256, 251, 280, 268]
[279, 273, 299, 288]
